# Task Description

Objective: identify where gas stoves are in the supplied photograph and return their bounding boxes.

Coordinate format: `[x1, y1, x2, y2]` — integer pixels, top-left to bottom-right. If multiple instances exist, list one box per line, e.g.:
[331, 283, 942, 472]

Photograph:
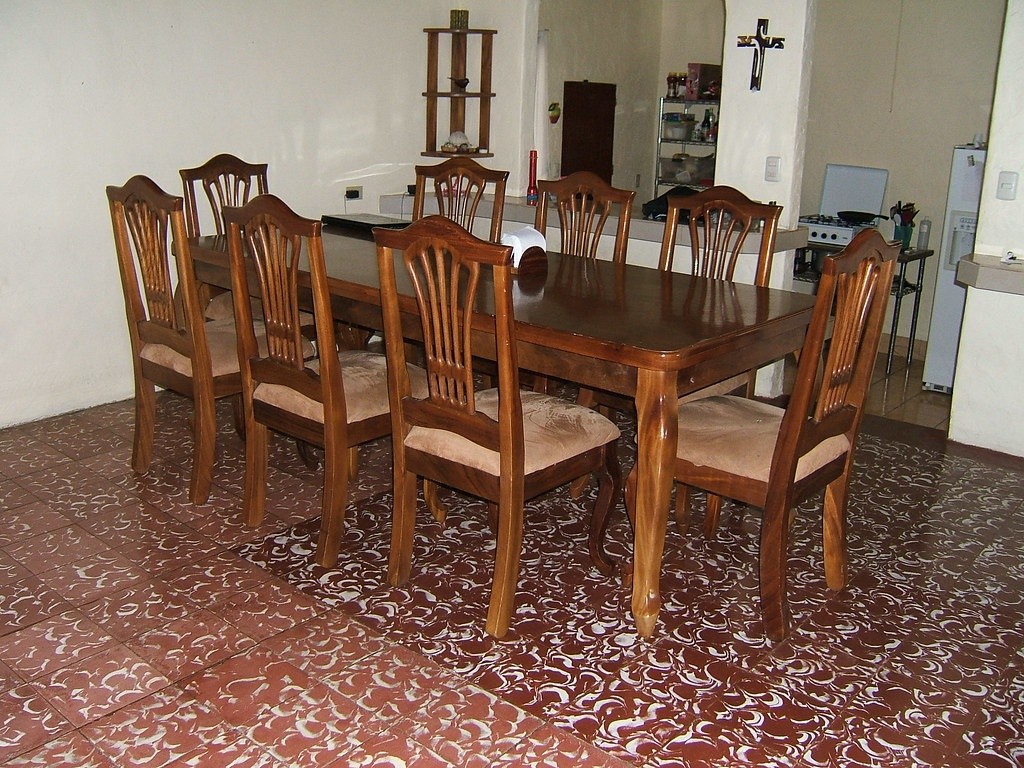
[797, 213, 874, 247]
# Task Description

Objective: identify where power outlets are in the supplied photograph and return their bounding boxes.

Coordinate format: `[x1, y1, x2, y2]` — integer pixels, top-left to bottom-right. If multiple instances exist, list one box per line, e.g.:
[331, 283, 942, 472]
[346, 186, 363, 200]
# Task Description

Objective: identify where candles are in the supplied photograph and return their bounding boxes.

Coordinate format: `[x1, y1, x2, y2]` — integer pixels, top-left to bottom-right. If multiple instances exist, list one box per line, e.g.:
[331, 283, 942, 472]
[669, 72, 676, 76]
[679, 73, 687, 77]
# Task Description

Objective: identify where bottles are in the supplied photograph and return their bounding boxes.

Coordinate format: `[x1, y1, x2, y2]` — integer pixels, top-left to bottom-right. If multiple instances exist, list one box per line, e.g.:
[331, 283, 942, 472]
[917, 216, 932, 251]
[709, 106, 715, 125]
[700, 109, 711, 142]
[666, 71, 677, 99]
[676, 71, 688, 98]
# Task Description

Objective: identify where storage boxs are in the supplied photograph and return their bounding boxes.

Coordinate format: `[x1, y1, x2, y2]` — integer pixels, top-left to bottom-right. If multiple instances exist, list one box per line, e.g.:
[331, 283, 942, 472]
[662, 120, 699, 141]
[686, 63, 722, 100]
[659, 158, 715, 184]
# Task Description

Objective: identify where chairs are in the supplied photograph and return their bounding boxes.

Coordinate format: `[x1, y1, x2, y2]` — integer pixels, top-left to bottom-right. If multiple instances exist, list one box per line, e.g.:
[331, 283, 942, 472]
[221, 194, 449, 568]
[483, 171, 636, 398]
[178, 153, 318, 342]
[402, 157, 510, 389]
[567, 185, 784, 537]
[372, 215, 622, 640]
[106, 175, 320, 506]
[624, 230, 903, 642]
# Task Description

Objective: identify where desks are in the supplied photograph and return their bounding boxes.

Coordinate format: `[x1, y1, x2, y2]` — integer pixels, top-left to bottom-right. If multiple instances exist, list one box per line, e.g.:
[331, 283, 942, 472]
[173, 224, 818, 638]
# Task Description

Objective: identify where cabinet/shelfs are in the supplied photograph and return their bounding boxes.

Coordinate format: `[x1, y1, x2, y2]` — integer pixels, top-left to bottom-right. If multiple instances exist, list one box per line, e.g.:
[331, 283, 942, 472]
[421, 28, 497, 158]
[654, 97, 721, 200]
[793, 242, 934, 374]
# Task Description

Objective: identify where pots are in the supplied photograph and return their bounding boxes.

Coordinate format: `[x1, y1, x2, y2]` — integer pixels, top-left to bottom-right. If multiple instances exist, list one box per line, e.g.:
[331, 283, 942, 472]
[837, 210, 890, 224]
